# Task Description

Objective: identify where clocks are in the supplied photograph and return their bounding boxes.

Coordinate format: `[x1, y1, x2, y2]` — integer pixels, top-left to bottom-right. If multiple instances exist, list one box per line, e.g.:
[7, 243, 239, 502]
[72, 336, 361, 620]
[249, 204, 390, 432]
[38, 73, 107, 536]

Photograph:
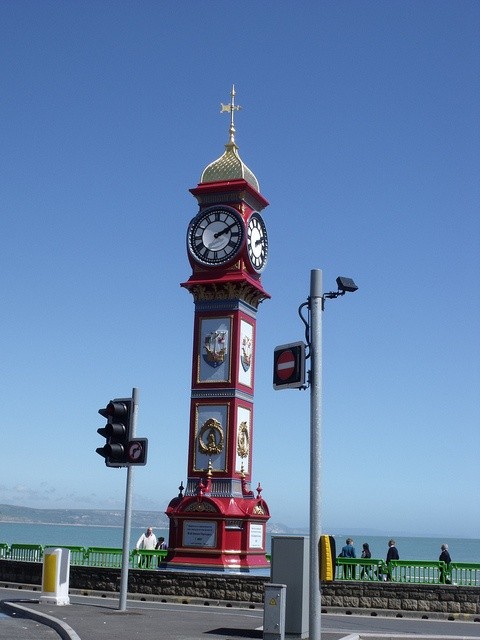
[186, 204, 247, 271]
[218, 239, 226, 241]
[243, 210, 269, 279]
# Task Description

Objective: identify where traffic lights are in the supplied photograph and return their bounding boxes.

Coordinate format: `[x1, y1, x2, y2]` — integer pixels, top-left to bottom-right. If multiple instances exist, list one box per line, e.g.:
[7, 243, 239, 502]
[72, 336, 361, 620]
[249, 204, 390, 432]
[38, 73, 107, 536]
[96, 397, 137, 469]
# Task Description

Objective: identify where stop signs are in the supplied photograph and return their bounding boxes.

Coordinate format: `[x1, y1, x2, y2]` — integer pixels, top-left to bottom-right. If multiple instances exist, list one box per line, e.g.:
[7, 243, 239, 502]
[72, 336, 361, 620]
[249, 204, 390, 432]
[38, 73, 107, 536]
[273, 341, 306, 391]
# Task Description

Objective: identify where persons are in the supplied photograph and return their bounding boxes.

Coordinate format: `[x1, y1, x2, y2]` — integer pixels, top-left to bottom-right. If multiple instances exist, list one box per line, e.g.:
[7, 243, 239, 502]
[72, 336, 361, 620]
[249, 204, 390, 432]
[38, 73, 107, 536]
[360, 543, 372, 580]
[342, 538, 357, 579]
[385, 540, 399, 581]
[134, 528, 158, 569]
[439, 544, 451, 584]
[154, 536, 168, 561]
